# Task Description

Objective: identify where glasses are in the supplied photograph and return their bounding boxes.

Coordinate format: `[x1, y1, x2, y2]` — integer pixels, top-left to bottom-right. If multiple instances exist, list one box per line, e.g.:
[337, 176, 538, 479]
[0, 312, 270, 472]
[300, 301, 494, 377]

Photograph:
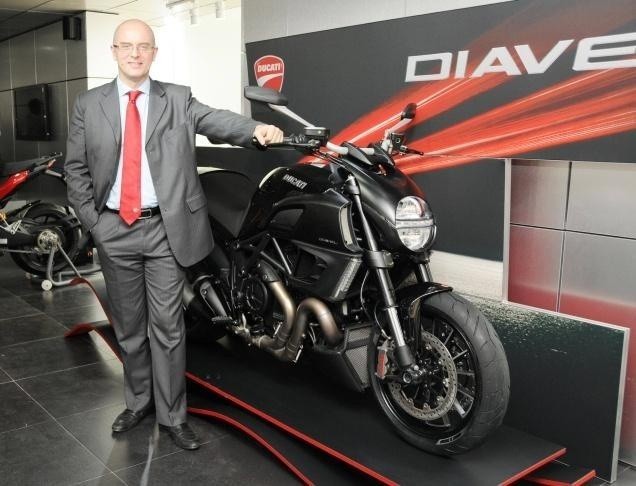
[114, 44, 156, 53]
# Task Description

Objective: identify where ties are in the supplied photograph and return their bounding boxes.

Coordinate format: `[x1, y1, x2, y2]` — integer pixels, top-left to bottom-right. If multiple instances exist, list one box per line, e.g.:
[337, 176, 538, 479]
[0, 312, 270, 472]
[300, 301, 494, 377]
[119, 91, 141, 225]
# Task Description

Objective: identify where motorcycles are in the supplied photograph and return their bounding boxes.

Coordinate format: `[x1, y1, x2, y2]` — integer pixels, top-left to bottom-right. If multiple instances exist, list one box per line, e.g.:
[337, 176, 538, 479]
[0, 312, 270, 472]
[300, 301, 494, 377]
[179, 84, 511, 459]
[0, 153, 83, 278]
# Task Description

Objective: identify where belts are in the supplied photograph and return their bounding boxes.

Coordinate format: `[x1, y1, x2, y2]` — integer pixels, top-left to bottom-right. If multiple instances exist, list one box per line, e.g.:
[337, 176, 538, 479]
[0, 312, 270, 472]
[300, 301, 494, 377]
[110, 205, 160, 220]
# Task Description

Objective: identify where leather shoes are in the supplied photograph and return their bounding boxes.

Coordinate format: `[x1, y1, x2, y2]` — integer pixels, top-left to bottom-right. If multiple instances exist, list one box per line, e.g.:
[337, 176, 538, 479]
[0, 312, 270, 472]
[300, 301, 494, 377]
[158, 422, 200, 450]
[112, 403, 153, 432]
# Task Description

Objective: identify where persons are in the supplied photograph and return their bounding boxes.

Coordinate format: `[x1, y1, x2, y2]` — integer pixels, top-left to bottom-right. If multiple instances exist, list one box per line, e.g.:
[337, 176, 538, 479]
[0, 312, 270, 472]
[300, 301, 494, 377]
[62, 17, 285, 450]
